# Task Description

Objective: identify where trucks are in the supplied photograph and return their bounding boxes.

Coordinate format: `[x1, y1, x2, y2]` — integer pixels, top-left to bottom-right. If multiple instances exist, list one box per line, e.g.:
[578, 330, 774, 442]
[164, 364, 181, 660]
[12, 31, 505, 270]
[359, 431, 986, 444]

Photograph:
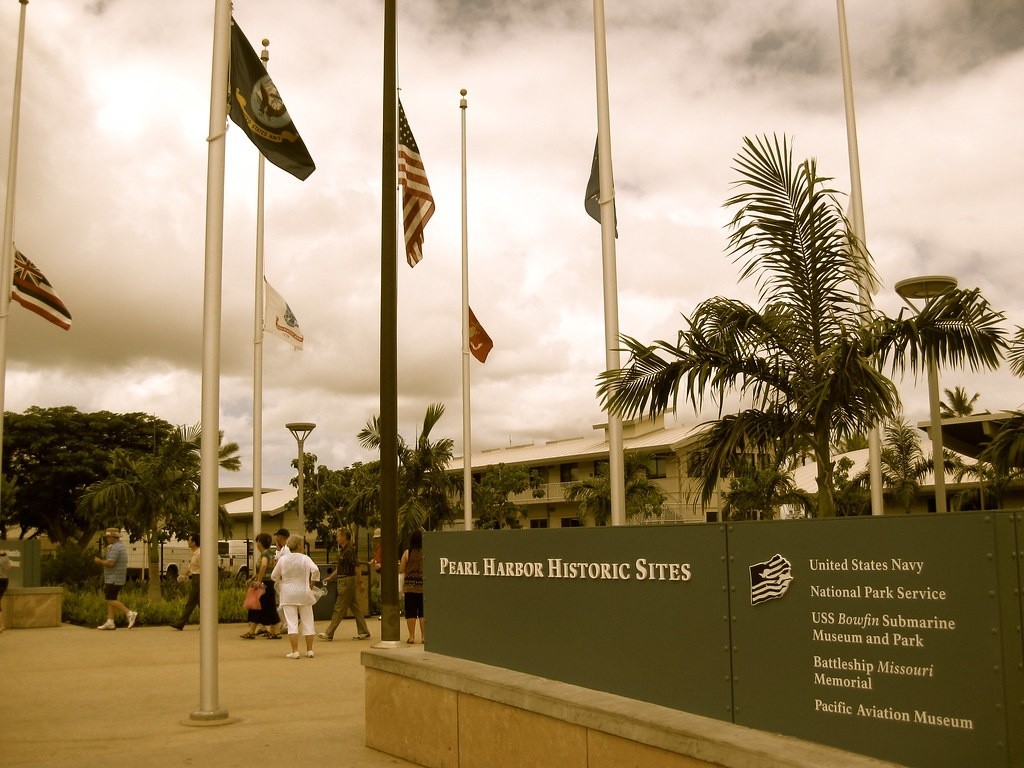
[104, 526, 224, 587]
[217, 539, 278, 583]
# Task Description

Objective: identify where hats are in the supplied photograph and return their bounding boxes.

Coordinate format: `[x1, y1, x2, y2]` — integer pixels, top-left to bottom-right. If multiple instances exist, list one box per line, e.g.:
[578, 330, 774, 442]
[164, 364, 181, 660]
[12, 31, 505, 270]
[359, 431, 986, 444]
[274, 528, 290, 536]
[103, 528, 122, 537]
[373, 528, 381, 537]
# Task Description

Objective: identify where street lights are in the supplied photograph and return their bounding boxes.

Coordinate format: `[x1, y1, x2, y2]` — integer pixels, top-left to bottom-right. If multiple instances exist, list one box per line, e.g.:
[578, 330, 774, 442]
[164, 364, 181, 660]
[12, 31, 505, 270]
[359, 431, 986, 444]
[285, 422, 317, 553]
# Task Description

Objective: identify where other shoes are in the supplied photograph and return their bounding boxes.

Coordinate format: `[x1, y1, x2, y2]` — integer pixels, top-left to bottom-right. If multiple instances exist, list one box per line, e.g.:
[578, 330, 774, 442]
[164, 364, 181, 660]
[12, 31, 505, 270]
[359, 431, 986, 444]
[128, 612, 138, 629]
[286, 650, 300, 659]
[407, 639, 414, 644]
[316, 632, 333, 641]
[97, 622, 116, 630]
[169, 623, 182, 630]
[353, 633, 370, 640]
[305, 651, 315, 658]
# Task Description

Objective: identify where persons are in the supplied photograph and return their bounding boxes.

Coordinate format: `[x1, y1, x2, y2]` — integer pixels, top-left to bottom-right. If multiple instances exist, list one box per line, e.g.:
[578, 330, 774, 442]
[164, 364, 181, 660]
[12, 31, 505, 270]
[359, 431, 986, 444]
[315, 527, 371, 641]
[240, 529, 292, 639]
[400, 531, 424, 644]
[271, 535, 320, 659]
[94, 528, 138, 630]
[371, 528, 382, 620]
[168, 534, 200, 630]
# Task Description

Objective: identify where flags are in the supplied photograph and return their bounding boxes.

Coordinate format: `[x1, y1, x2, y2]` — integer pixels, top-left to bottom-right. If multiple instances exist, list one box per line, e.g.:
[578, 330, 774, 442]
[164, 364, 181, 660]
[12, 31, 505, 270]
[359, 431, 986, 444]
[394, 100, 435, 267]
[263, 281, 304, 352]
[841, 195, 878, 295]
[10, 249, 72, 330]
[469, 306, 494, 363]
[225, 15, 316, 180]
[585, 134, 619, 239]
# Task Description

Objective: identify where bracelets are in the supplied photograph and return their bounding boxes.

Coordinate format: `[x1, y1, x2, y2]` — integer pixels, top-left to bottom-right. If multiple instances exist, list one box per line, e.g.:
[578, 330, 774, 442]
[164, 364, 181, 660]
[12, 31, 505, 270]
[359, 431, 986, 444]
[357, 580, 362, 583]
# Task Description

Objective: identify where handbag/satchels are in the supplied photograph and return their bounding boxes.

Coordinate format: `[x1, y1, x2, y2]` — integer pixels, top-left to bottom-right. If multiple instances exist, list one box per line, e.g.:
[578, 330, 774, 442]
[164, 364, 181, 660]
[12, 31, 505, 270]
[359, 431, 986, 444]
[243, 586, 261, 609]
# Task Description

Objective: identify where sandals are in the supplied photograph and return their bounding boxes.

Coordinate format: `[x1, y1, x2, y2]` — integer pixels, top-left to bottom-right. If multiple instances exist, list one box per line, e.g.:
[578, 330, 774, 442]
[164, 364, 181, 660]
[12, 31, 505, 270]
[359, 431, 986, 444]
[240, 632, 255, 639]
[255, 629, 268, 635]
[267, 633, 282, 639]
[262, 632, 272, 637]
